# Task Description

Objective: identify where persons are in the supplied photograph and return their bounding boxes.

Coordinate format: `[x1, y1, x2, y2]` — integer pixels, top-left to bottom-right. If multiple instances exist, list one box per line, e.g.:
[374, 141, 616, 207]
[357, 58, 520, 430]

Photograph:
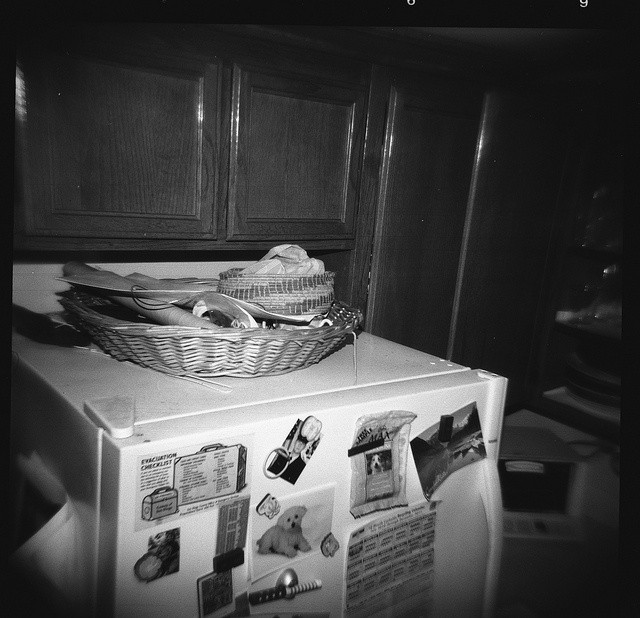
[270, 434, 310, 485]
[155, 530, 180, 579]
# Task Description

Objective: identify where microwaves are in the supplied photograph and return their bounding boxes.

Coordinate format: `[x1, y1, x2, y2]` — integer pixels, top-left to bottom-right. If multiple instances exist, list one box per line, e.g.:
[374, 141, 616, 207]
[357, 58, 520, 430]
[496, 426, 578, 544]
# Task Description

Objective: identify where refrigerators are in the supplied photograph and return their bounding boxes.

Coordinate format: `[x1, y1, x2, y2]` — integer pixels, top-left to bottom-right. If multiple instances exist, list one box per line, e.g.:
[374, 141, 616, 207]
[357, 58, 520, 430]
[1, 310, 509, 618]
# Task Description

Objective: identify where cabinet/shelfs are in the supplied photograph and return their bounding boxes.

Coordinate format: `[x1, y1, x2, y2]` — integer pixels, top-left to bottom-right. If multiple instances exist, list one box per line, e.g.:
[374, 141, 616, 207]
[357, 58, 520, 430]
[349, 74, 499, 370]
[13, 42, 367, 242]
